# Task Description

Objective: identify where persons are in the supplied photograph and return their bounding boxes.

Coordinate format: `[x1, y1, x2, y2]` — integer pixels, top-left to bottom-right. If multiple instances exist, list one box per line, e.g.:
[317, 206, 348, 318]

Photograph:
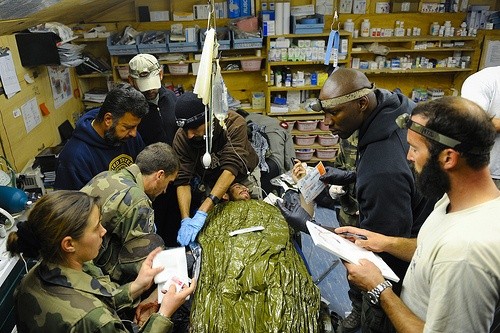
[134, 159, 320, 333]
[54, 84, 149, 190]
[462, 66, 500, 190]
[173, 92, 259, 247]
[346, 97, 500, 333]
[318, 69, 442, 333]
[6, 190, 196, 333]
[127, 53, 182, 248]
[78, 142, 180, 288]
[238, 109, 295, 196]
[329, 130, 362, 332]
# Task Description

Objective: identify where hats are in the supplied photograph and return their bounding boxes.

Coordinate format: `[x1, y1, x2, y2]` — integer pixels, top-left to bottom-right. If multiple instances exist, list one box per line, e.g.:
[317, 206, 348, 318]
[128, 54, 162, 92]
[174, 94, 210, 130]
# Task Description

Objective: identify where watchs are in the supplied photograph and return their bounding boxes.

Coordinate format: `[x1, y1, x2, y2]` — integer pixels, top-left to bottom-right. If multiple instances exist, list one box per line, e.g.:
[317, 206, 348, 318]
[367, 280, 392, 304]
[207, 194, 219, 205]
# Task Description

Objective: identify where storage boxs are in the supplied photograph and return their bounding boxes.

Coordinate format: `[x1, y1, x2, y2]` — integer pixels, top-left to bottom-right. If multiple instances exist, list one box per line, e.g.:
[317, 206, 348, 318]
[350, 42, 471, 70]
[259, 0, 469, 37]
[73, 25, 111, 38]
[107, 0, 266, 109]
[269, 37, 348, 160]
[411, 87, 445, 102]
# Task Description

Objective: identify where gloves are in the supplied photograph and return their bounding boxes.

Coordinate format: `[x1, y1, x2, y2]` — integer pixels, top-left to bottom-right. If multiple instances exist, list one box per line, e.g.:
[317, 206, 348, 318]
[276, 192, 322, 235]
[177, 210, 208, 247]
[319, 166, 356, 184]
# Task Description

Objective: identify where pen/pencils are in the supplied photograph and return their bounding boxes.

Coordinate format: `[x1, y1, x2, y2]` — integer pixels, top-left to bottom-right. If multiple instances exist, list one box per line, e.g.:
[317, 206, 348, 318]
[342, 232, 367, 240]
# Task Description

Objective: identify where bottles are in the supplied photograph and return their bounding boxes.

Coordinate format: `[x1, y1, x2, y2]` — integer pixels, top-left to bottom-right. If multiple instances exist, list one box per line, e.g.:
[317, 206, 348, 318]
[343, 18, 455, 37]
[400, 54, 452, 69]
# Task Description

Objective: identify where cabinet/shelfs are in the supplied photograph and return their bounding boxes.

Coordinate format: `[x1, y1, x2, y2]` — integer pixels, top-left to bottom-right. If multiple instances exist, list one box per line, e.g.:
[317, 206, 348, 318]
[349, 34, 482, 73]
[264, 29, 353, 168]
[111, 20, 269, 116]
[70, 35, 112, 110]
